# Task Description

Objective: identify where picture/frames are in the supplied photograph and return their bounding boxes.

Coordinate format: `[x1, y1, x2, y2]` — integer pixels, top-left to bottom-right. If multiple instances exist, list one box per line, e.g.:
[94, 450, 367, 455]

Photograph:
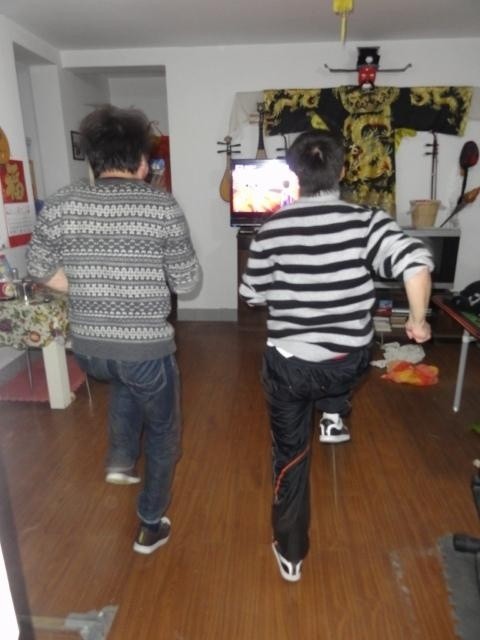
[71, 131, 85, 161]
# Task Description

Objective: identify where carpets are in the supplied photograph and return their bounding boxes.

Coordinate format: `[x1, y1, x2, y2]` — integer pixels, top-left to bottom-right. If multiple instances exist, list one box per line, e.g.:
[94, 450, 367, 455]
[438, 532, 480, 640]
[1, 357, 86, 402]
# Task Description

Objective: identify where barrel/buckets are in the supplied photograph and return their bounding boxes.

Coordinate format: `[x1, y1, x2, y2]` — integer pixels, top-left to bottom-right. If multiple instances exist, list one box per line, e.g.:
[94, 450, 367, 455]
[407, 200, 440, 229]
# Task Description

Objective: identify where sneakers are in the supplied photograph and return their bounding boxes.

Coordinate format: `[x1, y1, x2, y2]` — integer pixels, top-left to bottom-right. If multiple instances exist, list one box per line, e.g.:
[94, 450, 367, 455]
[319, 418, 350, 443]
[272, 539, 302, 582]
[106, 470, 141, 485]
[133, 516, 171, 555]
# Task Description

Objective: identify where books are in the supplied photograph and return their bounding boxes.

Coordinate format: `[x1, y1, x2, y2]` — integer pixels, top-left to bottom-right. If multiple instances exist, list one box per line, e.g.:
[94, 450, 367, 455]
[372, 307, 432, 332]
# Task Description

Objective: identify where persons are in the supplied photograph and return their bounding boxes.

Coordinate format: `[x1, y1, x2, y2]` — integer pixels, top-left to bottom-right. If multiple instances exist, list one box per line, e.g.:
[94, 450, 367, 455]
[232, 48, 474, 223]
[238, 129, 436, 582]
[26, 106, 204, 556]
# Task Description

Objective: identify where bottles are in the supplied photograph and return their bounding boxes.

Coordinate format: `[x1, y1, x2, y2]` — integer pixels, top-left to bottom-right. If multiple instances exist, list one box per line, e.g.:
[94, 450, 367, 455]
[0, 254, 17, 302]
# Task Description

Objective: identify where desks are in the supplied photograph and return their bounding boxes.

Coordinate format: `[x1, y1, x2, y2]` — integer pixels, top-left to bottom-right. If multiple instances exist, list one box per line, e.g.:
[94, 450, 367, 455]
[1, 281, 77, 411]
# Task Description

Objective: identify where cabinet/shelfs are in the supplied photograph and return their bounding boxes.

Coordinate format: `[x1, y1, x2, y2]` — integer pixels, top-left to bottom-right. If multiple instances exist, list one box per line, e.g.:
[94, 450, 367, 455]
[231, 218, 461, 339]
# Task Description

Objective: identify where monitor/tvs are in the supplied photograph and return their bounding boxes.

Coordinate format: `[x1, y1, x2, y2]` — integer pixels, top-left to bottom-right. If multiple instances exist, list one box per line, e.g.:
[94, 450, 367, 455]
[229, 158, 299, 233]
